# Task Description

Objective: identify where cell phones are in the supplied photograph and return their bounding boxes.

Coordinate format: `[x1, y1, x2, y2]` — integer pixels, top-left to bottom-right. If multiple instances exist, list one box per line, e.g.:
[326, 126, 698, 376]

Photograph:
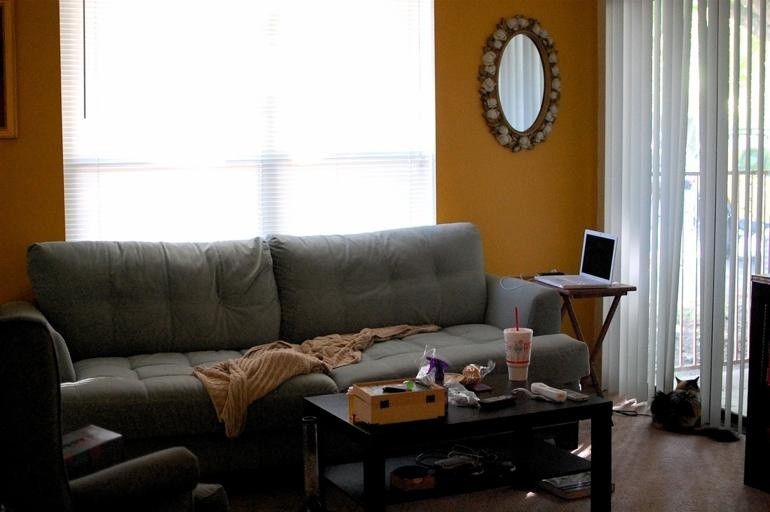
[561, 388, 591, 401]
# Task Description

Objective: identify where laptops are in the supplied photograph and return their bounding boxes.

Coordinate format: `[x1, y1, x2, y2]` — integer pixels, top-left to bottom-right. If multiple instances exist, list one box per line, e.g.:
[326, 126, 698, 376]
[534, 228, 618, 288]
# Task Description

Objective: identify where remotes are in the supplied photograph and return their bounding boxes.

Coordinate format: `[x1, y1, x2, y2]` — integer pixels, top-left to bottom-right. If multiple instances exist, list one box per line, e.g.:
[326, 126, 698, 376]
[477, 393, 518, 407]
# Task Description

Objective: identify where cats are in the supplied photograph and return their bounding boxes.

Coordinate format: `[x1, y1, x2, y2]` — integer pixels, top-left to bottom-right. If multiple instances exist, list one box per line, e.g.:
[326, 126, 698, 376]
[649, 376, 741, 443]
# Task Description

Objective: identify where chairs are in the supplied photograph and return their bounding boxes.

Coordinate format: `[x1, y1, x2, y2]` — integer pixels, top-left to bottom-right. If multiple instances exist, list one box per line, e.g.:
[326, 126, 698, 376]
[5, 298, 228, 512]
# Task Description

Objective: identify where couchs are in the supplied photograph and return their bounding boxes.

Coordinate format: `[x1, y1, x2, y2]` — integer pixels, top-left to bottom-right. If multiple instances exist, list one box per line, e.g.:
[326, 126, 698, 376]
[2, 222, 593, 512]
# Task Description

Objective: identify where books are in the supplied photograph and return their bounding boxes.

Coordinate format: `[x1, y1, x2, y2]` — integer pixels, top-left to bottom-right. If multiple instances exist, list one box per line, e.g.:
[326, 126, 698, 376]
[538, 470, 615, 501]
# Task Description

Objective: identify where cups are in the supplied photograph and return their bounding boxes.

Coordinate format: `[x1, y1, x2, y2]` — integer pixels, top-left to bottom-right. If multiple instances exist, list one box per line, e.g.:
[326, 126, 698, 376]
[503, 327, 533, 381]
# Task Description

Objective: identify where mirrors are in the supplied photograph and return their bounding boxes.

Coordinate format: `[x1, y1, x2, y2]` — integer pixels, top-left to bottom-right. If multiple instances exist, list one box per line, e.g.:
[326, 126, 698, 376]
[478, 14, 562, 154]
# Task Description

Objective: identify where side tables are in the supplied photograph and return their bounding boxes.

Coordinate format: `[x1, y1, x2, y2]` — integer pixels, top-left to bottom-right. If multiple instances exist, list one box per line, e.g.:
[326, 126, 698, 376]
[529, 279, 637, 402]
